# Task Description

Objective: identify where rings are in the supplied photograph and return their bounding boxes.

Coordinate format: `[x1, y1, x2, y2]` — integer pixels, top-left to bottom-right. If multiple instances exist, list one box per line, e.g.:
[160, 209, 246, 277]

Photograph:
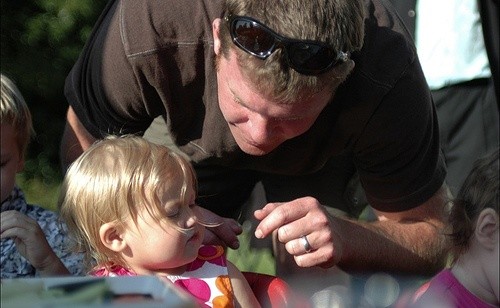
[301, 235, 312, 253]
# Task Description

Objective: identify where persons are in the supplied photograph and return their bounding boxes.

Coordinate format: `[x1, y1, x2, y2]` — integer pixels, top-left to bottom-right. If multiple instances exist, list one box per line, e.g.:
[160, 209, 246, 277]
[362, 0, 500, 199]
[0, 74, 86, 276]
[62, 1, 456, 275]
[62, 133, 261, 308]
[390, 143, 500, 307]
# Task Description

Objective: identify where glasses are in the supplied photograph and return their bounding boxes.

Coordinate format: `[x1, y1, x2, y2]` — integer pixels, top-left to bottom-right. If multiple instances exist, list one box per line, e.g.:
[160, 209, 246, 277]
[225, 14, 355, 75]
